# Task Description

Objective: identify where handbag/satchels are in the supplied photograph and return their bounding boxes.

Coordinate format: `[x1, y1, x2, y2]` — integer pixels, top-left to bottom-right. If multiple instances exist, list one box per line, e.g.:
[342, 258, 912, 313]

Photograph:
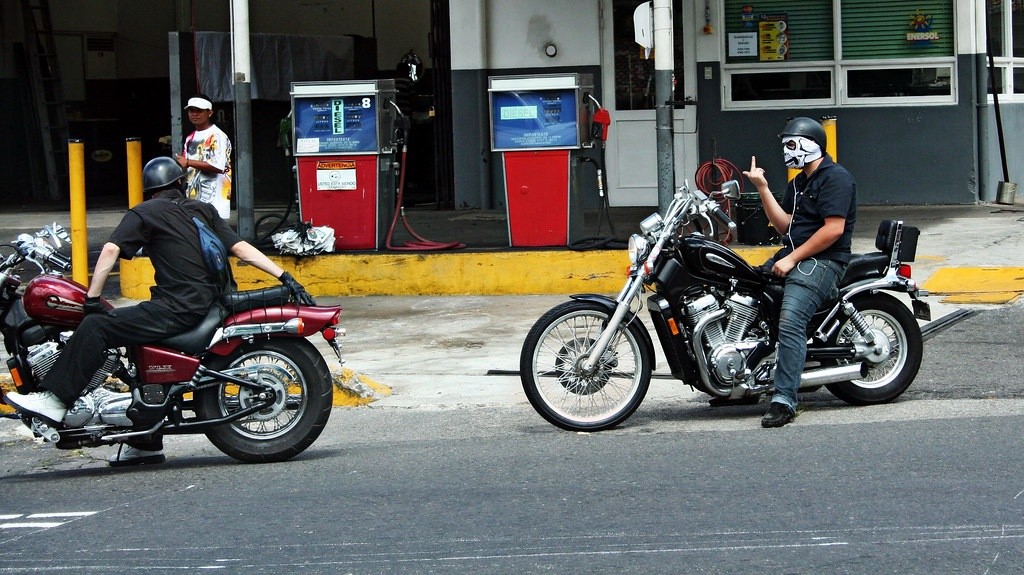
[192, 215, 227, 275]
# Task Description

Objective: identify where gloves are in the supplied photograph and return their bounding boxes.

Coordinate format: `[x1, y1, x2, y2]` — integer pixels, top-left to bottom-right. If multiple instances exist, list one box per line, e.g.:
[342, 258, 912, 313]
[278, 270, 316, 306]
[83, 294, 119, 317]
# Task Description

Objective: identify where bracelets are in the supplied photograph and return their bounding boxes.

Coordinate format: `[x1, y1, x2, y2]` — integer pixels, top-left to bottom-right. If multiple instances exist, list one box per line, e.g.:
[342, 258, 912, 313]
[186, 158, 189, 168]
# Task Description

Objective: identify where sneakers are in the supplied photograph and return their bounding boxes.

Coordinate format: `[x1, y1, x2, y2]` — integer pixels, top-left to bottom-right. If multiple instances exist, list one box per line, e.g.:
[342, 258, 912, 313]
[709, 394, 759, 407]
[109, 445, 165, 467]
[761, 402, 795, 427]
[3, 392, 67, 428]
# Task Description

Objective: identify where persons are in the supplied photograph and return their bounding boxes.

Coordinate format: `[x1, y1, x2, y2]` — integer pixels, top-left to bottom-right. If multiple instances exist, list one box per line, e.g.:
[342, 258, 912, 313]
[176, 94, 232, 222]
[3, 156, 315, 467]
[708, 117, 857, 427]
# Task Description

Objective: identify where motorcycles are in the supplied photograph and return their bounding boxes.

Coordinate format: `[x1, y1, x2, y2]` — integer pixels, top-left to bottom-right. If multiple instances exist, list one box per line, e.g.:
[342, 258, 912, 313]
[0, 220, 344, 464]
[518, 178, 931, 432]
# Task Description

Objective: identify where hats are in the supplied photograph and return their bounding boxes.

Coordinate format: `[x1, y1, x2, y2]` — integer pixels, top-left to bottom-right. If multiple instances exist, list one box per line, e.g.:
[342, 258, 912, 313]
[184, 97, 212, 109]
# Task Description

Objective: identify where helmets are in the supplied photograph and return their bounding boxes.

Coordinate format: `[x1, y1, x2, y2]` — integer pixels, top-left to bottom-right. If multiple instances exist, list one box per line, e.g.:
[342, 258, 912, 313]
[777, 117, 826, 152]
[142, 157, 184, 191]
